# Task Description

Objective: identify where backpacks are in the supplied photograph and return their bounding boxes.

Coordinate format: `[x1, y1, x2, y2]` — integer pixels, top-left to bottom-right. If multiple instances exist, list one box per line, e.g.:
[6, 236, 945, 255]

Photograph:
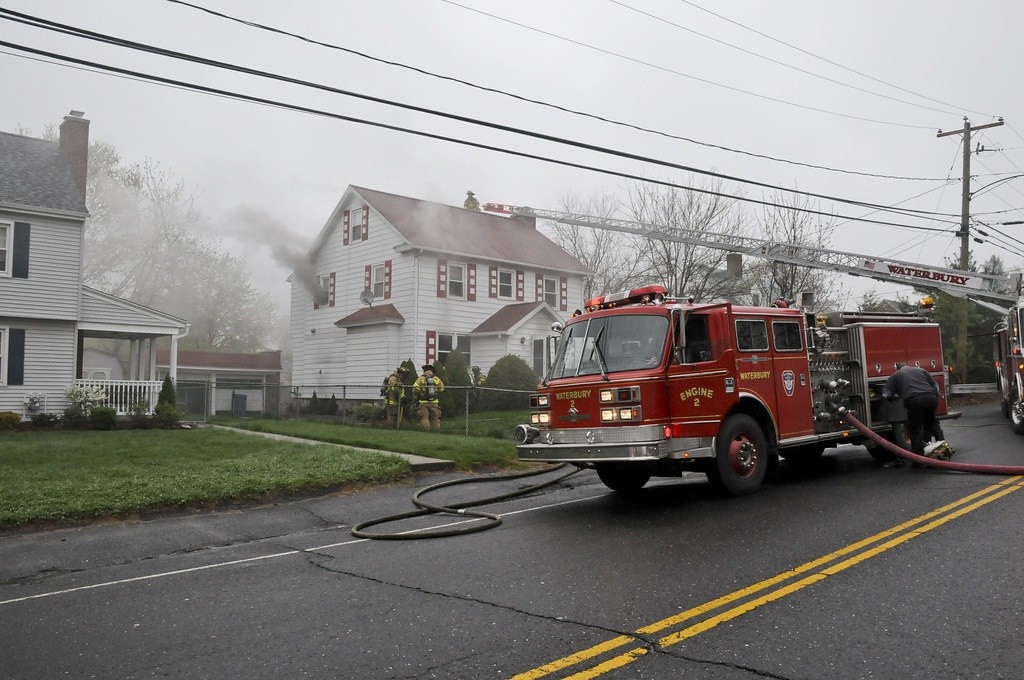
[380, 375, 399, 396]
[419, 375, 439, 401]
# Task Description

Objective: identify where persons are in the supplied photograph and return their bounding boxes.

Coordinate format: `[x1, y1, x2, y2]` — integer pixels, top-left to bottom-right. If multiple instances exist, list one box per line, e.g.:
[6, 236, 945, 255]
[385, 366, 411, 427]
[464, 191, 481, 211]
[882, 363, 955, 467]
[413, 364, 444, 430]
[472, 366, 487, 411]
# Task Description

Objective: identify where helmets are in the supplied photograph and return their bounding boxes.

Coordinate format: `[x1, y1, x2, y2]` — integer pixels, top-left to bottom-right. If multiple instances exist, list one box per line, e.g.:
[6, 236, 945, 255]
[397, 367, 410, 375]
[422, 365, 436, 374]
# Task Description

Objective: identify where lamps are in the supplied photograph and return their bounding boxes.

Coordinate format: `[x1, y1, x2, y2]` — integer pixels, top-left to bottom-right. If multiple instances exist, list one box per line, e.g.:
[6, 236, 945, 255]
[520, 336, 526, 344]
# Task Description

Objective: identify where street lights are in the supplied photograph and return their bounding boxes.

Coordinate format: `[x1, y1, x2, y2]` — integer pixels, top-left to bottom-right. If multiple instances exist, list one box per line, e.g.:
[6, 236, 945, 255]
[959, 218, 1024, 383]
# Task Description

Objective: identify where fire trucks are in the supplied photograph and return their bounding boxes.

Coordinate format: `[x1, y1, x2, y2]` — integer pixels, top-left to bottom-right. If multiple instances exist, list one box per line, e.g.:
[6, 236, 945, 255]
[515, 284, 961, 496]
[989, 299, 1024, 435]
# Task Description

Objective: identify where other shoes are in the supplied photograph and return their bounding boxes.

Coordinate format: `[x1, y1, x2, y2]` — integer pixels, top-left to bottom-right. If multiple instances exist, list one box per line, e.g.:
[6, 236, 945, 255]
[911, 462, 921, 469]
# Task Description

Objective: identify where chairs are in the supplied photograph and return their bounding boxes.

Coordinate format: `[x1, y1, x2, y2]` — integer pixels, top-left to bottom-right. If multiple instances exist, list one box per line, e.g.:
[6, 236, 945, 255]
[686, 319, 705, 363]
